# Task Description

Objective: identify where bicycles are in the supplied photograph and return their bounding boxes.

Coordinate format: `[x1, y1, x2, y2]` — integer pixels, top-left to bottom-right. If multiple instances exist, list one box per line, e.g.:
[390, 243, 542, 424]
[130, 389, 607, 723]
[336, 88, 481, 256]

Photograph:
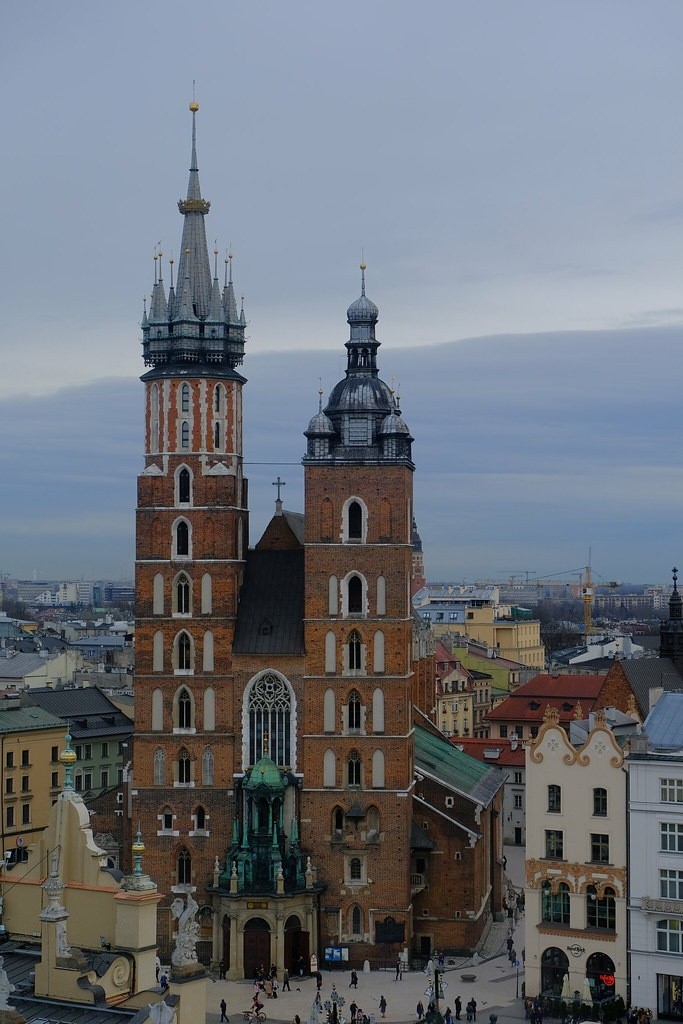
[242, 1005, 266, 1022]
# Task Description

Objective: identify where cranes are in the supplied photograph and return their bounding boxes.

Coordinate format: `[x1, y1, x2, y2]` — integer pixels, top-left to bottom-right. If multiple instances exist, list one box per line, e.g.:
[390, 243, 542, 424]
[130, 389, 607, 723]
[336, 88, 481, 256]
[474, 547, 624, 644]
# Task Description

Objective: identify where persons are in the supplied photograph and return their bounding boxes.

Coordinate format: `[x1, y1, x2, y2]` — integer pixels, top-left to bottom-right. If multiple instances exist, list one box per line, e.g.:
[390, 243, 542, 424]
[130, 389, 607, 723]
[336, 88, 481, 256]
[348, 969, 358, 989]
[503, 855, 507, 871]
[417, 995, 477, 1024]
[298, 955, 306, 976]
[251, 962, 280, 1001]
[507, 934, 526, 968]
[160, 971, 170, 989]
[424, 951, 446, 976]
[315, 991, 322, 1013]
[283, 968, 291, 992]
[220, 998, 229, 1023]
[218, 958, 228, 979]
[316, 971, 323, 990]
[523, 983, 653, 1024]
[350, 1000, 359, 1019]
[379, 995, 387, 1018]
[502, 887, 525, 916]
[396, 958, 403, 981]
